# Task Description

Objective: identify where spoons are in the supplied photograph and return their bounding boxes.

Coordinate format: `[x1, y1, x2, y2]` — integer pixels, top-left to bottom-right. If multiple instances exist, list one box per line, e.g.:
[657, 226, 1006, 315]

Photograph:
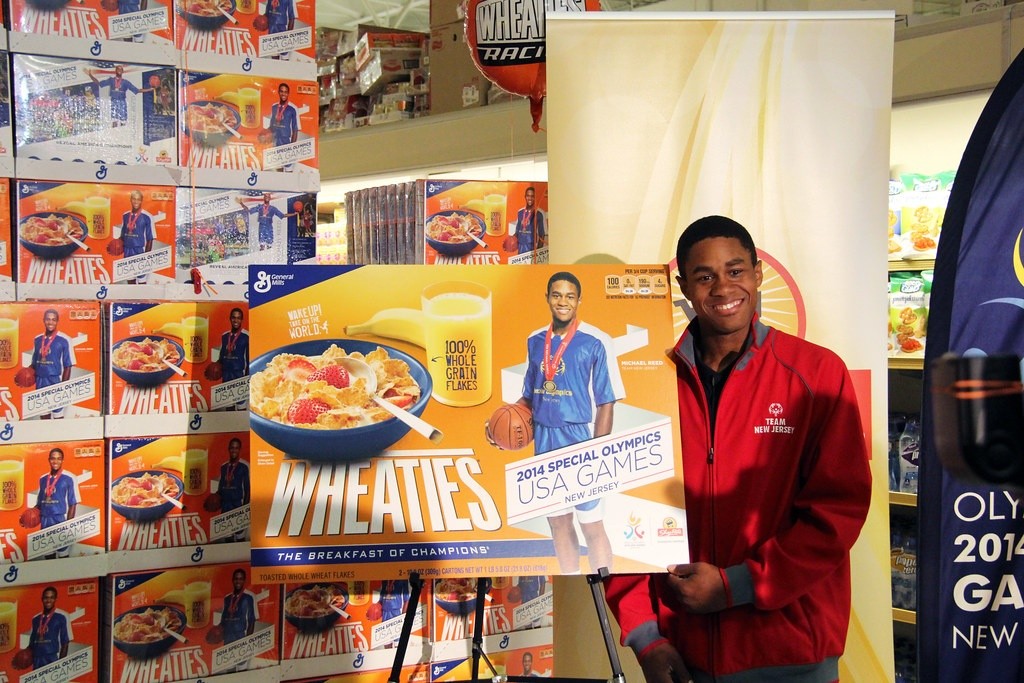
[146, 477, 187, 512]
[147, 612, 188, 645]
[455, 217, 488, 251]
[61, 225, 90, 252]
[216, 111, 242, 139]
[331, 357, 443, 444]
[147, 342, 187, 378]
[318, 589, 352, 620]
[215, 0, 238, 24]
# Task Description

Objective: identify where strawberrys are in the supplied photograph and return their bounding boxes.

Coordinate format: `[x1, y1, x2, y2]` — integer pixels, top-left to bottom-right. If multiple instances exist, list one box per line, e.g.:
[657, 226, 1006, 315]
[283, 359, 412, 424]
[299, 591, 342, 615]
[126, 479, 176, 506]
[436, 216, 481, 242]
[189, 4, 230, 17]
[125, 342, 177, 370]
[447, 579, 478, 600]
[34, 218, 81, 244]
[127, 615, 179, 643]
[194, 107, 234, 132]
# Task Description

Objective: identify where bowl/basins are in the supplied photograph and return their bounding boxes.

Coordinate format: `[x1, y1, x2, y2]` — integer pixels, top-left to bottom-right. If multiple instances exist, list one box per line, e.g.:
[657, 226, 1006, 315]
[176, 0, 236, 30]
[285, 583, 350, 632]
[25, 0, 70, 11]
[180, 100, 241, 148]
[249, 339, 433, 461]
[424, 210, 487, 257]
[110, 469, 184, 522]
[20, 211, 89, 259]
[113, 605, 186, 658]
[112, 334, 186, 387]
[435, 577, 492, 616]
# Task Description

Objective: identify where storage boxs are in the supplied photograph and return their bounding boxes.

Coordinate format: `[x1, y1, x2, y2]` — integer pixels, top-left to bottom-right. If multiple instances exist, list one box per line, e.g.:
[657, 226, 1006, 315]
[2, 0, 531, 682]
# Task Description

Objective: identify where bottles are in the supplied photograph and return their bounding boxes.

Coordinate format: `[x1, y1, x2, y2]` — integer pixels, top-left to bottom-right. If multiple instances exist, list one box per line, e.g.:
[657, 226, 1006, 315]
[888, 414, 920, 680]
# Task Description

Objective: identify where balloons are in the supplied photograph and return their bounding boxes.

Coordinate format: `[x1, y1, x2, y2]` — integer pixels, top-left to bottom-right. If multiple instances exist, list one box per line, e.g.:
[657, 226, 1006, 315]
[464, 0, 602, 132]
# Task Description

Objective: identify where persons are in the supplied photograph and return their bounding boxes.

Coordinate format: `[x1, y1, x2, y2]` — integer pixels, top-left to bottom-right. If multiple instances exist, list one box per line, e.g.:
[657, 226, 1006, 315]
[602, 215, 873, 683]
[265, 0, 298, 61]
[84, 66, 159, 129]
[213, 438, 250, 544]
[519, 652, 541, 677]
[365, 579, 412, 650]
[516, 576, 548, 630]
[217, 569, 259, 673]
[235, 193, 302, 251]
[14, 310, 77, 420]
[267, 83, 301, 172]
[103, 0, 148, 44]
[203, 308, 250, 411]
[106, 190, 157, 286]
[299, 202, 316, 237]
[502, 187, 548, 254]
[154, 77, 174, 116]
[18, 448, 81, 560]
[484, 272, 627, 573]
[12, 586, 74, 671]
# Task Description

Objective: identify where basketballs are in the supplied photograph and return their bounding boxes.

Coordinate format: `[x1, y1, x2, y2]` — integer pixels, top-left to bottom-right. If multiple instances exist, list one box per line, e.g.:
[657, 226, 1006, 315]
[293, 201, 303, 211]
[366, 603, 382, 621]
[20, 507, 42, 529]
[100, 0, 119, 11]
[257, 129, 272, 145]
[507, 587, 521, 604]
[108, 239, 124, 256]
[205, 626, 224, 645]
[148, 75, 160, 87]
[205, 362, 221, 381]
[252, 15, 268, 32]
[13, 650, 33, 669]
[487, 403, 534, 450]
[203, 493, 222, 513]
[16, 367, 35, 387]
[503, 236, 518, 252]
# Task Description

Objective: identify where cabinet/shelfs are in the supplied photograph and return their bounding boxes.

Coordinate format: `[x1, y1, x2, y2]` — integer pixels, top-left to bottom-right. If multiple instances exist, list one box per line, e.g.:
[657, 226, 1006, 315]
[887, 260, 938, 624]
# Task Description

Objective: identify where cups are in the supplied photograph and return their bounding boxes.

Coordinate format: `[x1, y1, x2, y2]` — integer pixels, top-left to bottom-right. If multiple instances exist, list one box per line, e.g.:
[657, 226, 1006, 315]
[180, 311, 209, 363]
[483, 190, 507, 236]
[85, 193, 110, 239]
[237, 83, 261, 129]
[181, 444, 209, 496]
[0, 596, 17, 652]
[419, 280, 493, 407]
[347, 581, 370, 605]
[1, 313, 19, 369]
[184, 575, 211, 628]
[0, 454, 24, 510]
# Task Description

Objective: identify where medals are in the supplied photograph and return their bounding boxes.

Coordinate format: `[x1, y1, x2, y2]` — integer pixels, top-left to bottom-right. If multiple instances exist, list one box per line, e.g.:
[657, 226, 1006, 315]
[543, 381, 556, 392]
[523, 229, 526, 233]
